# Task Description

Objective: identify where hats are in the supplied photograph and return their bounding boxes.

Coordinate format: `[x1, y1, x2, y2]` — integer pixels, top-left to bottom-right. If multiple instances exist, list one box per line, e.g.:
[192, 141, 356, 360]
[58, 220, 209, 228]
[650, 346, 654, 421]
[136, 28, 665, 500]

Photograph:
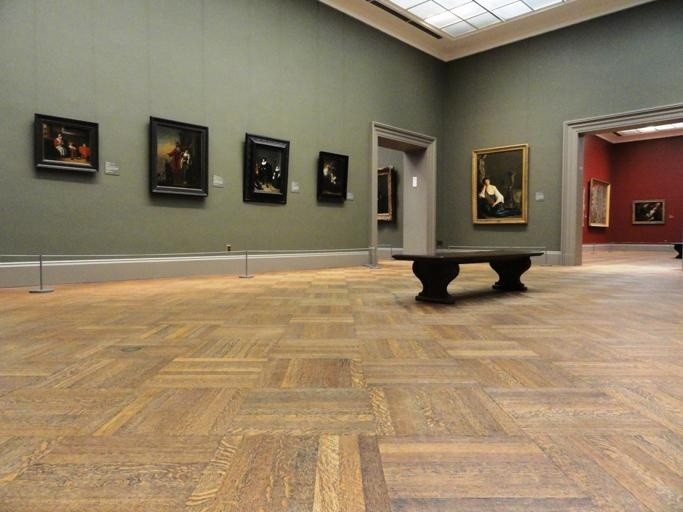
[260, 157, 268, 162]
[176, 142, 180, 146]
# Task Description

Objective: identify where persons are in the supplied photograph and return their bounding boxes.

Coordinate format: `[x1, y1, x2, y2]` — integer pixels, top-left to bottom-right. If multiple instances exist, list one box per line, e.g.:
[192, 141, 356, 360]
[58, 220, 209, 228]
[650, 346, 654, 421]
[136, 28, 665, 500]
[478, 178, 520, 217]
[166, 142, 192, 184]
[54, 133, 65, 158]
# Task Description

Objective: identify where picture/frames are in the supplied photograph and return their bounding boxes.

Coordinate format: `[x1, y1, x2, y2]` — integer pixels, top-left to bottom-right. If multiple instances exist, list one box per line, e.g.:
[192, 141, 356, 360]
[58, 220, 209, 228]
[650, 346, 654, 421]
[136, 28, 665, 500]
[375, 162, 395, 222]
[149, 116, 209, 199]
[316, 151, 349, 203]
[632, 198, 667, 227]
[243, 132, 290, 205]
[587, 175, 613, 229]
[469, 141, 528, 227]
[33, 113, 99, 175]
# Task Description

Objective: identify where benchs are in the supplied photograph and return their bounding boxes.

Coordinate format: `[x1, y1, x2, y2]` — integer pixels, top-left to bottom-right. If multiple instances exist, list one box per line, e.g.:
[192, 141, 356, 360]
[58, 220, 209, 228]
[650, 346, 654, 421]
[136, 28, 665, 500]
[390, 249, 547, 304]
